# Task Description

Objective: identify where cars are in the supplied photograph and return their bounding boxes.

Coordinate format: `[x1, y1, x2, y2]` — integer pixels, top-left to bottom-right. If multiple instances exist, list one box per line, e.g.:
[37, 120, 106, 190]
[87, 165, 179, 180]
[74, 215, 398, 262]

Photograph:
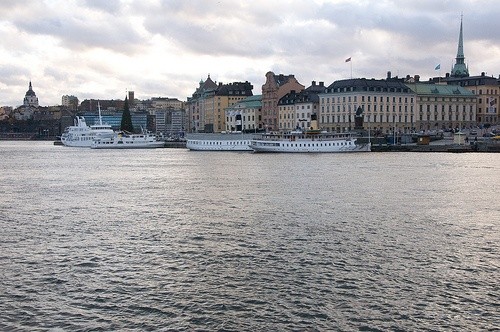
[483, 132, 495, 137]
[470, 129, 477, 134]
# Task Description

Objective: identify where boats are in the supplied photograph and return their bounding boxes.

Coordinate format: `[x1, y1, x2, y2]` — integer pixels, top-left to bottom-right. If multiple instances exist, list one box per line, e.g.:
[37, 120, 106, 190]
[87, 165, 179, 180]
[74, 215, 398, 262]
[247, 133, 370, 153]
[92, 133, 164, 148]
[185, 130, 276, 150]
[60, 102, 114, 147]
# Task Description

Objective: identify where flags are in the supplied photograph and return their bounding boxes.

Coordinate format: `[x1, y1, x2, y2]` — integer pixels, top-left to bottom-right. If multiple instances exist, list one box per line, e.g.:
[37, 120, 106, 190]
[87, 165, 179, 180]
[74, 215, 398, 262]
[435, 63, 440, 69]
[345, 58, 351, 62]
